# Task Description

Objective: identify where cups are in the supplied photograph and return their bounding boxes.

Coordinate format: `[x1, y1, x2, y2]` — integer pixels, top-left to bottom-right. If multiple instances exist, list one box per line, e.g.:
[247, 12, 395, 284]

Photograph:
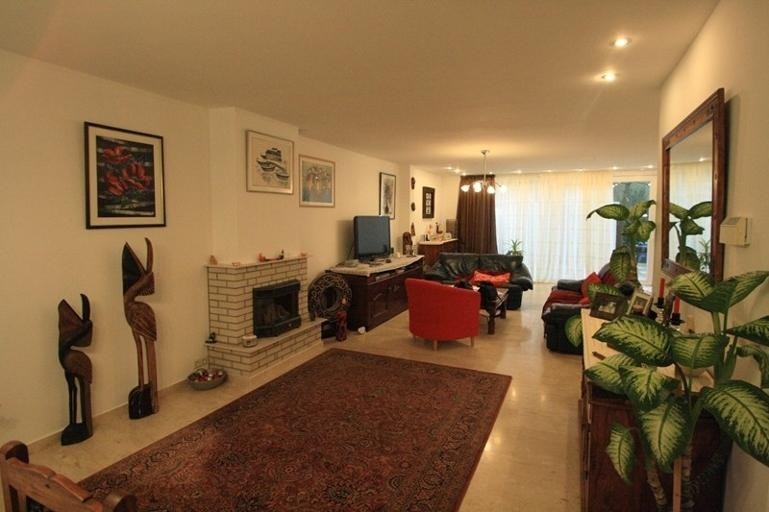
[420, 234, 425, 241]
[405, 245, 410, 257]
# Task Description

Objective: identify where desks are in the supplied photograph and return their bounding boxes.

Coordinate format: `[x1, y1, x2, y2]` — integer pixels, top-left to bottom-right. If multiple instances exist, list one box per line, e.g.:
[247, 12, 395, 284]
[417, 239, 458, 268]
[332, 254, 424, 332]
[580, 301, 731, 512]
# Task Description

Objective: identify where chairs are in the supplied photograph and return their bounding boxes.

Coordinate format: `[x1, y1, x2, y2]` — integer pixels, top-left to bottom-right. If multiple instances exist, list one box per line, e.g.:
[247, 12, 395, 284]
[0, 440, 136, 512]
[405, 278, 481, 352]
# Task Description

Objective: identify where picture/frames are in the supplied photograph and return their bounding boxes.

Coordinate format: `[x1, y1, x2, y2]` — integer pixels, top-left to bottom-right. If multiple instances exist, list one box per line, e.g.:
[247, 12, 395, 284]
[297, 154, 336, 207]
[83, 121, 166, 228]
[246, 129, 295, 195]
[422, 185, 434, 218]
[380, 171, 396, 219]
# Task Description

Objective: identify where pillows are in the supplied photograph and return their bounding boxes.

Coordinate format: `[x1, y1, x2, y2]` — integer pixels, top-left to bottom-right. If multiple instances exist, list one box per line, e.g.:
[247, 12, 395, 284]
[578, 296, 591, 304]
[472, 270, 510, 285]
[581, 272, 600, 294]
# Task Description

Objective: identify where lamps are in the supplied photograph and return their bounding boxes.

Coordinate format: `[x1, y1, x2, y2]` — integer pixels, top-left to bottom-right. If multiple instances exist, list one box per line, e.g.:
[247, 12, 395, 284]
[460, 149, 507, 193]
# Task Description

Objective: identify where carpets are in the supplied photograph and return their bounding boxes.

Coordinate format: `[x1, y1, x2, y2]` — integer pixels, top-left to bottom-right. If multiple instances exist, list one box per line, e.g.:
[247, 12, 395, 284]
[74, 347, 512, 512]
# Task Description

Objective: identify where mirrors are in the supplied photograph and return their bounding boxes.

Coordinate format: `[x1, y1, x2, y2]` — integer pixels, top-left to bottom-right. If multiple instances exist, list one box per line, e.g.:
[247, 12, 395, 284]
[661, 86, 724, 311]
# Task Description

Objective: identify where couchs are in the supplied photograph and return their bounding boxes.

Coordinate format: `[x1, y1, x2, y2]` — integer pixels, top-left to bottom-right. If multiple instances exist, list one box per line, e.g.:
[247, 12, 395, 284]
[424, 251, 533, 309]
[541, 258, 644, 355]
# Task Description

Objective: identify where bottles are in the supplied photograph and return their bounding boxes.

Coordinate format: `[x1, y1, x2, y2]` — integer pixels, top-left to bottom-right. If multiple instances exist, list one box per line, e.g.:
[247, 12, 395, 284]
[412, 245, 416, 257]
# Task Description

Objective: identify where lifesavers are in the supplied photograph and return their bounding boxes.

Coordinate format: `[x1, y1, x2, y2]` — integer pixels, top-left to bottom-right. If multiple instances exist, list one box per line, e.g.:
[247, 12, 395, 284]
[307, 273, 352, 324]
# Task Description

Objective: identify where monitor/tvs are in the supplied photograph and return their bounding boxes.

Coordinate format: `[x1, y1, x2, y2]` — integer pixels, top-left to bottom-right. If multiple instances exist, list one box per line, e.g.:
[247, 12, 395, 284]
[353, 215, 390, 261]
